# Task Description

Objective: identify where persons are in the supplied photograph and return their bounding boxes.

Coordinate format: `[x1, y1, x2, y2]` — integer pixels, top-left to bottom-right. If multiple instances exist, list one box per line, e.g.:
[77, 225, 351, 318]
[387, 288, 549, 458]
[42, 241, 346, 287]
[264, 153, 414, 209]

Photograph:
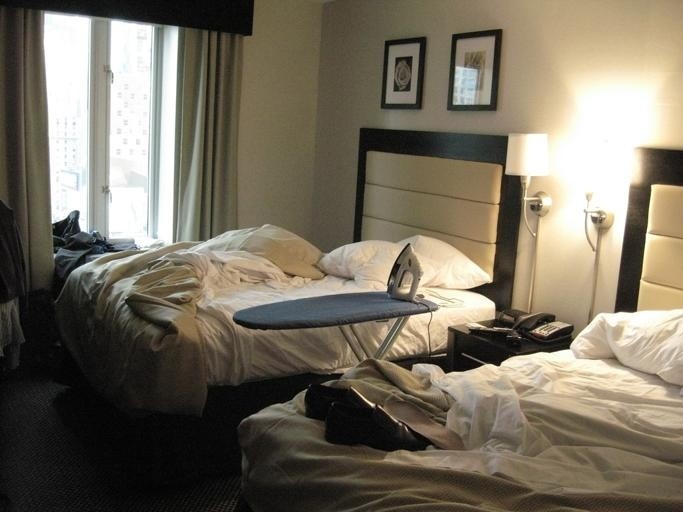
[0, 199, 28, 380]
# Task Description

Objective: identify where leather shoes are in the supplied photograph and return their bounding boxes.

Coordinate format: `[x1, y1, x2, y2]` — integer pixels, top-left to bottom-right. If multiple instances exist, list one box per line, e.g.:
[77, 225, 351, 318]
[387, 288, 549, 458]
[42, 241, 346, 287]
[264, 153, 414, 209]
[305, 383, 370, 420]
[324, 401, 432, 451]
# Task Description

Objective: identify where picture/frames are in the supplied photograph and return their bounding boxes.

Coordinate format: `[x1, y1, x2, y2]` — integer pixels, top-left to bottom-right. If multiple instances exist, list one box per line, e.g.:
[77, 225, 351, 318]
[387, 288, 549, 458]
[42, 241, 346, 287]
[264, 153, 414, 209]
[378, 29, 503, 112]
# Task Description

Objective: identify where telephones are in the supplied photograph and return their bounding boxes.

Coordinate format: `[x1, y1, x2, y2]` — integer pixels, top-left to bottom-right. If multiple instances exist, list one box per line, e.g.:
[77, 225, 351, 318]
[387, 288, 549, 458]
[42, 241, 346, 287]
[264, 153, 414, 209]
[520, 312, 574, 346]
